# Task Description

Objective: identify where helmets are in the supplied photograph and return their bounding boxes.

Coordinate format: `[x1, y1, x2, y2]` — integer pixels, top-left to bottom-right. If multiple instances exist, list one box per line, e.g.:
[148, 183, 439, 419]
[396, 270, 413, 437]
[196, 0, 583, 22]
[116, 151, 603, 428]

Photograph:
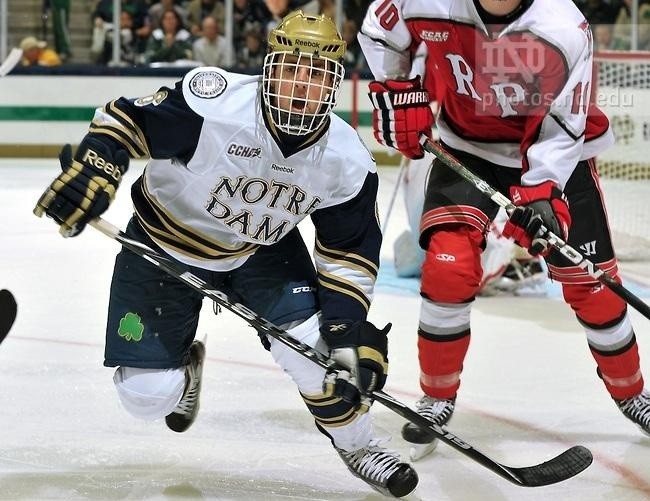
[262, 9, 346, 135]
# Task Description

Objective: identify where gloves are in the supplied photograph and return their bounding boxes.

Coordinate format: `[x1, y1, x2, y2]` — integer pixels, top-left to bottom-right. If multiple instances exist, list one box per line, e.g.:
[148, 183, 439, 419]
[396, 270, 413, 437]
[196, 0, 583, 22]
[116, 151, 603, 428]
[320, 319, 391, 414]
[502, 180, 572, 257]
[368, 75, 434, 159]
[33, 138, 129, 238]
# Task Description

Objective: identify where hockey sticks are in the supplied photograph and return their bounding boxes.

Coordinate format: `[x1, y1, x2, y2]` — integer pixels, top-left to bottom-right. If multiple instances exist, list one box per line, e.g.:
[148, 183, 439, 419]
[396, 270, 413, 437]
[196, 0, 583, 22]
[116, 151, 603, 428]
[86, 215, 594, 488]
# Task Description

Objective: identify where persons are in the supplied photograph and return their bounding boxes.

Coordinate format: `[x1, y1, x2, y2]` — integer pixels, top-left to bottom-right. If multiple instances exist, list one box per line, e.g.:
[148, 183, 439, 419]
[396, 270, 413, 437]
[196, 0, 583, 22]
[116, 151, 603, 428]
[18, 36, 63, 66]
[572, 0, 650, 50]
[357, 0, 650, 444]
[89, 0, 374, 80]
[31, 9, 421, 498]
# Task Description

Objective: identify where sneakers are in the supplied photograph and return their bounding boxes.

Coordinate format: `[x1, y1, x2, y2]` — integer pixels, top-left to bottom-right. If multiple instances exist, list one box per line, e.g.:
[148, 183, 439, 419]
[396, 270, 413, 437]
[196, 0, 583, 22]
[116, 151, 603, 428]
[614, 389, 650, 433]
[500, 262, 542, 288]
[165, 340, 205, 432]
[401, 395, 455, 443]
[336, 440, 417, 497]
